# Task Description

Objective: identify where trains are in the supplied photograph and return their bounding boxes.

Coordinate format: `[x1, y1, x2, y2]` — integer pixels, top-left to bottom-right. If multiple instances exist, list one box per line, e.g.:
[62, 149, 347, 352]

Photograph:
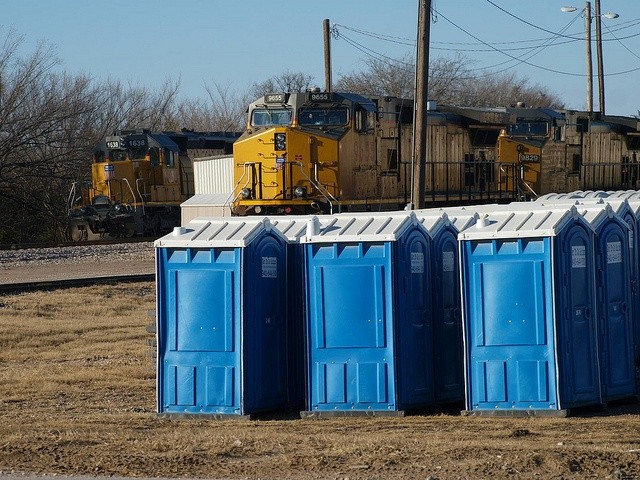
[64, 128, 241, 238]
[230, 88, 638, 214]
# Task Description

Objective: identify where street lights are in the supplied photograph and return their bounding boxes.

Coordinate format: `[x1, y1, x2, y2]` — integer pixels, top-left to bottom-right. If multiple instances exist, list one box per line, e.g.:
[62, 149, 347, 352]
[560, 5, 621, 115]
